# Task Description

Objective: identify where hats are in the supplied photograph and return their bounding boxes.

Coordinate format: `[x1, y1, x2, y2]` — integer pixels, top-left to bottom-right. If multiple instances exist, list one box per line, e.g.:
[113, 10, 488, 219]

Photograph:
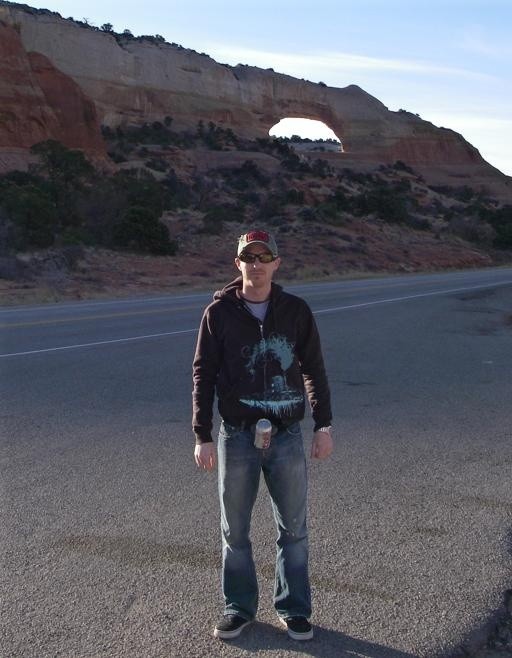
[236, 230, 279, 259]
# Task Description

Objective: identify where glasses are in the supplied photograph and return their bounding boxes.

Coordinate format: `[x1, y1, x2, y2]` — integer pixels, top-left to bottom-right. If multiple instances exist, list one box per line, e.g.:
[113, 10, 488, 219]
[238, 252, 277, 264]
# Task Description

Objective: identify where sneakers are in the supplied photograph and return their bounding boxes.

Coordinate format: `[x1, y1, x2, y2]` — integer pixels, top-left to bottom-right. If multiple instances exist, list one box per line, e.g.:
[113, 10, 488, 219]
[213, 613, 254, 640]
[276, 610, 314, 642]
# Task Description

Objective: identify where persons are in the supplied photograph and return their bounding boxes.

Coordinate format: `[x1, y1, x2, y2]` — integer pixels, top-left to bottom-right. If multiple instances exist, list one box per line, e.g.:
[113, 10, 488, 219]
[191, 230, 333, 640]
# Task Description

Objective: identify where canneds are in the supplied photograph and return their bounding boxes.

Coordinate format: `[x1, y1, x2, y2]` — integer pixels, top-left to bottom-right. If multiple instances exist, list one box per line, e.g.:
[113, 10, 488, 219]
[254, 419, 272, 449]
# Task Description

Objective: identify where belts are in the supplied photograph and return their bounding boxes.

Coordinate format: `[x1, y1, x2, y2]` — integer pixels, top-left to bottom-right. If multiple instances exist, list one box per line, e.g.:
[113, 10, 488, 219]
[222, 414, 300, 436]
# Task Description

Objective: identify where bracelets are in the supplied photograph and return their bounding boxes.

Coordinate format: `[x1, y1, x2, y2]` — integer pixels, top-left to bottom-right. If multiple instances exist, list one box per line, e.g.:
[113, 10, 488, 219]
[318, 425, 333, 435]
